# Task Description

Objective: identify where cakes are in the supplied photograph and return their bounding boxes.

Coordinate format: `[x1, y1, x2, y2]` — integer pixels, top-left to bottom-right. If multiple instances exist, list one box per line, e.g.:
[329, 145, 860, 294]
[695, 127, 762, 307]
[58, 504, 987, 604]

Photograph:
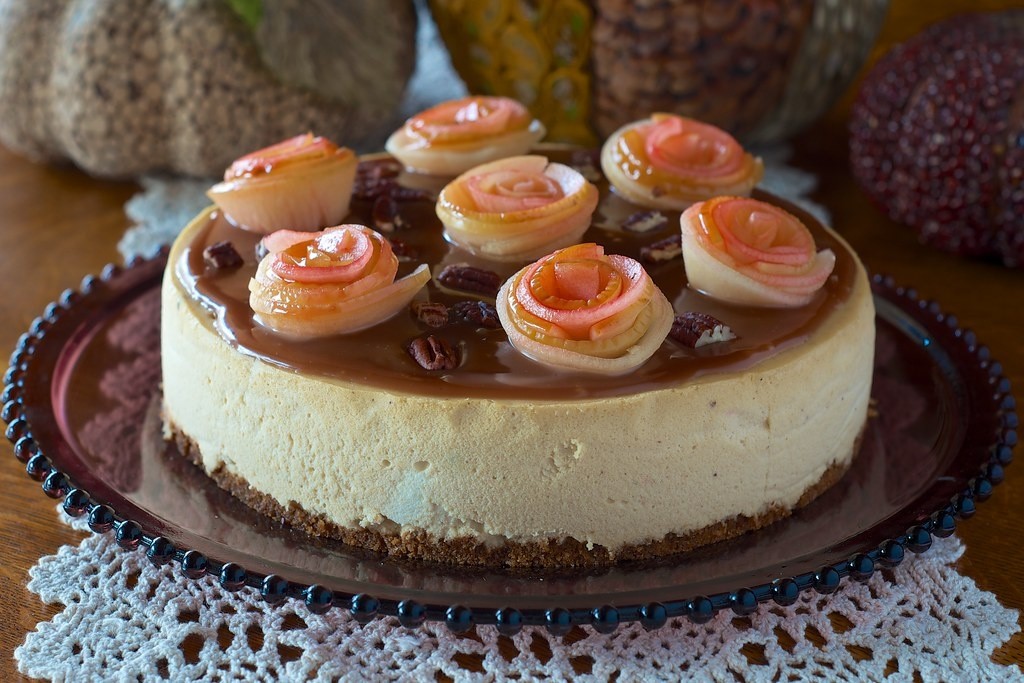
[159, 94, 876, 578]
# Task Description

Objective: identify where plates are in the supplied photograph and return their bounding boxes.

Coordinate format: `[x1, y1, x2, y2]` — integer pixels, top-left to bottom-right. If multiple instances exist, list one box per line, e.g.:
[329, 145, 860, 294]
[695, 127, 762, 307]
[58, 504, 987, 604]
[0, 245, 1019, 632]
